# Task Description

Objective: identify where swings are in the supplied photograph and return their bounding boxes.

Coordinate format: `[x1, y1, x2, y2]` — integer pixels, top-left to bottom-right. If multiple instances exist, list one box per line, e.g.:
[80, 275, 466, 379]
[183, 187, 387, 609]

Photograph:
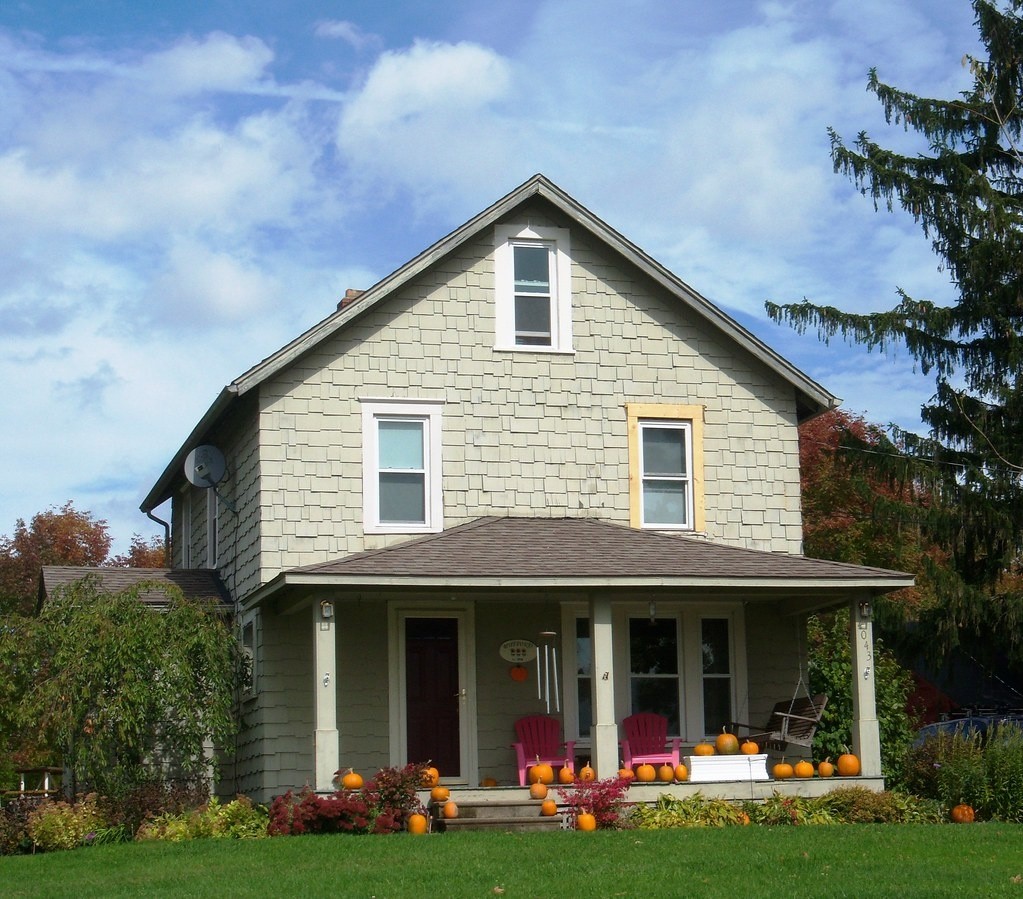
[725, 614, 828, 747]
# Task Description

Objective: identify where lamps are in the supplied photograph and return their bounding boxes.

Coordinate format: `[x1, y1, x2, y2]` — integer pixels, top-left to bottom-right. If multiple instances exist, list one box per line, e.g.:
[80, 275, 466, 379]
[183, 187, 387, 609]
[648, 594, 657, 621]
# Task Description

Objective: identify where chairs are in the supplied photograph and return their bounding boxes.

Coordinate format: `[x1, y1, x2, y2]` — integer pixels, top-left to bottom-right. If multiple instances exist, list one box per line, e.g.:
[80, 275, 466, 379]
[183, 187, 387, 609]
[618, 711, 681, 778]
[509, 715, 576, 786]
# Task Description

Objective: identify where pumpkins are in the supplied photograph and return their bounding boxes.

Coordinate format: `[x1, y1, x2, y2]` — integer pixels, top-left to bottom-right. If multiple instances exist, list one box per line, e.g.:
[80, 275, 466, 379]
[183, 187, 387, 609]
[530, 776, 547, 799]
[951, 804, 974, 823]
[542, 798, 557, 816]
[419, 765, 439, 787]
[343, 768, 364, 789]
[619, 759, 688, 782]
[430, 782, 449, 801]
[529, 754, 595, 784]
[443, 797, 458, 819]
[577, 808, 596, 831]
[772, 744, 860, 778]
[407, 812, 427, 834]
[481, 778, 496, 786]
[693, 725, 759, 755]
